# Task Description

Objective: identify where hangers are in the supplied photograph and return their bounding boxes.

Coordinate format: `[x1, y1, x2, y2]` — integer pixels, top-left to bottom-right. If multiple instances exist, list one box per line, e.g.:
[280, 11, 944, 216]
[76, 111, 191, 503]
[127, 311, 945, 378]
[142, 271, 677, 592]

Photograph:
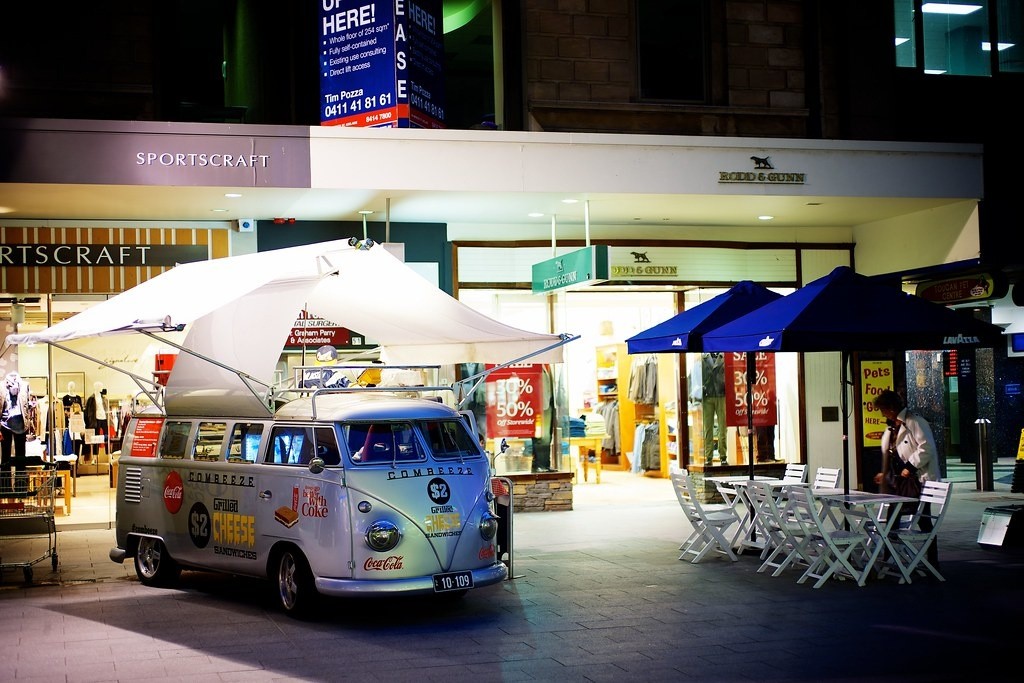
[603, 396, 619, 404]
[640, 415, 659, 425]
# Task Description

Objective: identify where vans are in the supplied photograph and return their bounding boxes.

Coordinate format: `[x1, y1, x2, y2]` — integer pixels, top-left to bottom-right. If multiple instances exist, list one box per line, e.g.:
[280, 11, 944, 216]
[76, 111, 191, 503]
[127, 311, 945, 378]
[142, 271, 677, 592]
[109, 363, 509, 622]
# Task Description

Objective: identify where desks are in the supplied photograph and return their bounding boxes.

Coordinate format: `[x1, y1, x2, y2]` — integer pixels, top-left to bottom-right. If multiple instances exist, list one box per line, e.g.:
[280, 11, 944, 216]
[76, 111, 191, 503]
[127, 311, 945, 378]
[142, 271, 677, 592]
[32, 470, 71, 514]
[564, 433, 610, 484]
[701, 476, 779, 549]
[784, 488, 865, 586]
[728, 480, 810, 553]
[824, 493, 920, 587]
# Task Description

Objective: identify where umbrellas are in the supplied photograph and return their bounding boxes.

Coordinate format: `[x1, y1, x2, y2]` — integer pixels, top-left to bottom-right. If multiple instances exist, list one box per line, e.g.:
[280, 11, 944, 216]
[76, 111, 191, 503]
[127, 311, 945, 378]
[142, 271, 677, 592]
[624, 280, 786, 542]
[700, 267, 1006, 565]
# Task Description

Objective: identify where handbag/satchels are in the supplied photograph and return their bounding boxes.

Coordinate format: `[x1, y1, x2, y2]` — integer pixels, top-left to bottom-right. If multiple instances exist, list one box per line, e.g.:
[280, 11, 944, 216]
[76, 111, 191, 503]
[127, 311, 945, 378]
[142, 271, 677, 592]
[892, 473, 919, 512]
[689, 386, 707, 402]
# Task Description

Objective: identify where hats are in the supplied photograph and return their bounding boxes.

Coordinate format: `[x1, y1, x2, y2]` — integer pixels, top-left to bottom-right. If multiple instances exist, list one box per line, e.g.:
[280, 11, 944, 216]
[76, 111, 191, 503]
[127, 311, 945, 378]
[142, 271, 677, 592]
[315, 344, 338, 366]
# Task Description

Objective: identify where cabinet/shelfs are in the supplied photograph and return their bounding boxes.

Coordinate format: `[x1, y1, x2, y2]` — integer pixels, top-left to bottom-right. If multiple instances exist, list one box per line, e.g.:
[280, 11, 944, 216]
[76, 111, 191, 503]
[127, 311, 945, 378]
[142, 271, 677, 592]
[595, 343, 635, 472]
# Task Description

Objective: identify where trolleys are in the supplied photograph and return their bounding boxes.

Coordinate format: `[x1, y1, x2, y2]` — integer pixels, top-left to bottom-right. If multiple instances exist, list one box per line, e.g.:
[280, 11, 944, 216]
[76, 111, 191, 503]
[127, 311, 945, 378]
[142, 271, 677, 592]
[0, 454, 60, 582]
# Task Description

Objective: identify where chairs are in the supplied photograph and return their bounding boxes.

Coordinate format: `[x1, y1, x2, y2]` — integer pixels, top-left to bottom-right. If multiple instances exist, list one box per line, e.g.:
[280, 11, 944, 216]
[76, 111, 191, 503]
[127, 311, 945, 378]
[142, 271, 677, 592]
[670, 461, 954, 588]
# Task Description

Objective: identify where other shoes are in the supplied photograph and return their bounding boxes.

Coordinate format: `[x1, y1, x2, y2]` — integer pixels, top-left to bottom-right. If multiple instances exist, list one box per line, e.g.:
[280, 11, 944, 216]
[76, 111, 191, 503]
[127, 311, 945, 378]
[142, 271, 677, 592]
[721, 457, 727, 465]
[705, 460, 712, 465]
[921, 561, 938, 571]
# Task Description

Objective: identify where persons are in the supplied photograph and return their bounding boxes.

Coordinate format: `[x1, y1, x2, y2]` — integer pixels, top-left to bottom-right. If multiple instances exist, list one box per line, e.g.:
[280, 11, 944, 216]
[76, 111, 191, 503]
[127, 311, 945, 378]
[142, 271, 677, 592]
[63, 382, 84, 465]
[0, 372, 30, 492]
[755, 427, 785, 463]
[86, 382, 112, 465]
[873, 390, 941, 576]
[688, 352, 728, 465]
[531, 364, 558, 472]
